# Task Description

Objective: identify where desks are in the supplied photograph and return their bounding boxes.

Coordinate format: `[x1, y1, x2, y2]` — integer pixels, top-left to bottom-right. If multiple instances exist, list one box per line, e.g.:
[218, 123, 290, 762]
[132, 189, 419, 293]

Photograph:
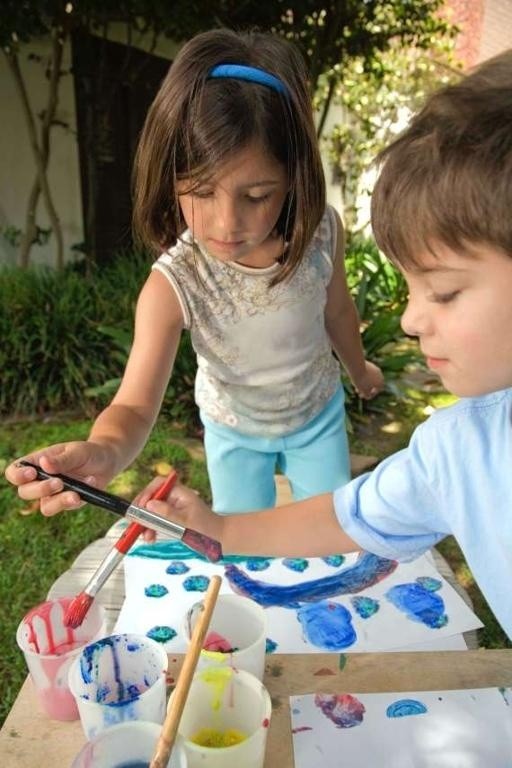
[44, 515, 479, 652]
[0, 648, 512, 768]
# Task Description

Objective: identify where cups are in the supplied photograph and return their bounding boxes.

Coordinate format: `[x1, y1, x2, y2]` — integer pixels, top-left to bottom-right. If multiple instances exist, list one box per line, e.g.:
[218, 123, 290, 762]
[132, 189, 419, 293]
[182, 593, 266, 682]
[67, 633, 169, 740]
[15, 598, 108, 720]
[165, 667, 272, 768]
[70, 720, 188, 768]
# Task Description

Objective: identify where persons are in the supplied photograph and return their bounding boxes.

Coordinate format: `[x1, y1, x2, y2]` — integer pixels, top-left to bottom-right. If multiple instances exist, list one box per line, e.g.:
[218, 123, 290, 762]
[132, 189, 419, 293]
[130, 47, 512, 645]
[4, 27, 385, 517]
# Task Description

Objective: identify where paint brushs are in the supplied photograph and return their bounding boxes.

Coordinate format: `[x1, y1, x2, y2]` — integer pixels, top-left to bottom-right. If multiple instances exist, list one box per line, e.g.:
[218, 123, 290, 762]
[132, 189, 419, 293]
[64, 468, 177, 629]
[17, 461, 222, 565]
